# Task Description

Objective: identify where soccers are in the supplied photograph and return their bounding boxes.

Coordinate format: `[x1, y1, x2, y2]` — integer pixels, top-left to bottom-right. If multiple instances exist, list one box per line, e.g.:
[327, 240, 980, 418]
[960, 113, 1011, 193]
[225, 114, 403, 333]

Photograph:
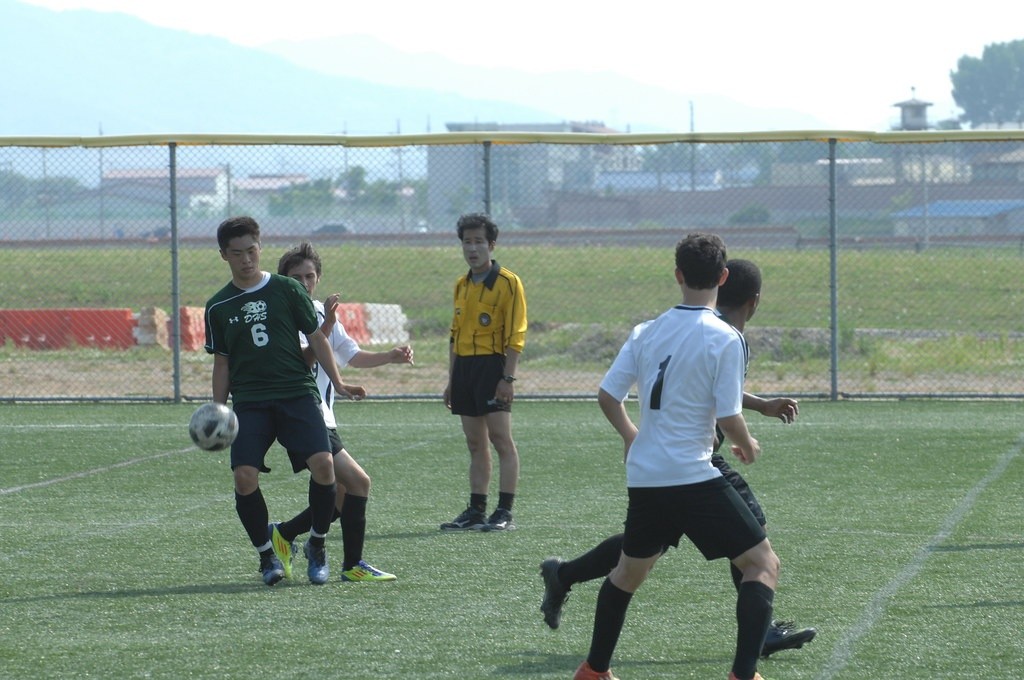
[189, 403, 239, 453]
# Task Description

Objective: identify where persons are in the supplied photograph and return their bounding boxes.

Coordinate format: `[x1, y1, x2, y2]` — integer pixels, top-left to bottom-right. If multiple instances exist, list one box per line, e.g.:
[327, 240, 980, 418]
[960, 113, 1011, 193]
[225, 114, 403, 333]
[540, 259, 816, 657]
[267, 242, 414, 581]
[203, 217, 366, 585]
[573, 234, 780, 680]
[441, 215, 526, 530]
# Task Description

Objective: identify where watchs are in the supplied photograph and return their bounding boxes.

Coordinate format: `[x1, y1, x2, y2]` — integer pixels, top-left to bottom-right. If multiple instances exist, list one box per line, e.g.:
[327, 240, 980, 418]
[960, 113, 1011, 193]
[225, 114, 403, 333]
[502, 375, 516, 382]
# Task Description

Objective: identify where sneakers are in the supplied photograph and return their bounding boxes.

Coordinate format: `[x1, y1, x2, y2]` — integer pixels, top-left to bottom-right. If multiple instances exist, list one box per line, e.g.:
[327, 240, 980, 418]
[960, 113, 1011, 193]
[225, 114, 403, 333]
[540, 556, 571, 629]
[729, 671, 764, 680]
[760, 619, 816, 657]
[440, 502, 489, 531]
[259, 554, 285, 587]
[268, 522, 298, 579]
[573, 660, 620, 680]
[340, 559, 397, 582]
[303, 539, 329, 585]
[481, 508, 516, 532]
